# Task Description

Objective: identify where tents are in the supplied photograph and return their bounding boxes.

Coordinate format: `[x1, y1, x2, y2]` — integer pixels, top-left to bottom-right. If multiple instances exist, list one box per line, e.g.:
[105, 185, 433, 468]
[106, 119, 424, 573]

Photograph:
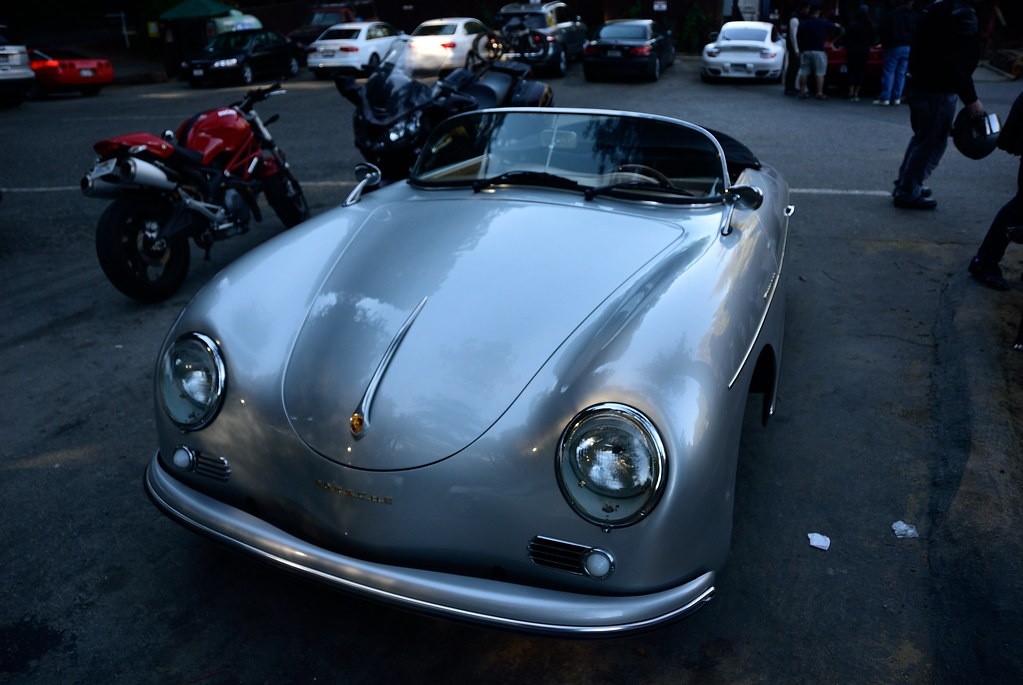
[159, 0, 232, 46]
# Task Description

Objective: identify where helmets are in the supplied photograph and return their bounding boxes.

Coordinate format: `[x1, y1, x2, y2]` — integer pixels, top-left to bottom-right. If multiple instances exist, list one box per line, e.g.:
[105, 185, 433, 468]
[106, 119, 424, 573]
[954, 106, 1003, 160]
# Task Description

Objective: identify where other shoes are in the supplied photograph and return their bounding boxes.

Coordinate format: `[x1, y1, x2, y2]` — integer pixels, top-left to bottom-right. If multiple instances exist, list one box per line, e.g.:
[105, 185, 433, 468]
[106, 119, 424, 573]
[892, 99, 900, 106]
[799, 91, 812, 99]
[872, 99, 889, 105]
[849, 94, 860, 102]
[816, 93, 832, 99]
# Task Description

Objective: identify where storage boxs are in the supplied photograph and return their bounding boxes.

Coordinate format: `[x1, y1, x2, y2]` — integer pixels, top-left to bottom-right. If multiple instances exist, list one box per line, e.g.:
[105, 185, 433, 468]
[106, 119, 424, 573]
[506, 81, 553, 140]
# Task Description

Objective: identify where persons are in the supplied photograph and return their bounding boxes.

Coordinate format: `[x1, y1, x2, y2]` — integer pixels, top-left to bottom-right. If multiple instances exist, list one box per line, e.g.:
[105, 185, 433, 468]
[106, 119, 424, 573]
[965, 90, 1023, 290]
[892, 0, 985, 211]
[785, 0, 912, 105]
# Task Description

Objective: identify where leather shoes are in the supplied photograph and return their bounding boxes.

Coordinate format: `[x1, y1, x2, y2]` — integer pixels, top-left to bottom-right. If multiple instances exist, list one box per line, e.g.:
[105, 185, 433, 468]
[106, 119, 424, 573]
[893, 187, 938, 211]
[968, 256, 1011, 292]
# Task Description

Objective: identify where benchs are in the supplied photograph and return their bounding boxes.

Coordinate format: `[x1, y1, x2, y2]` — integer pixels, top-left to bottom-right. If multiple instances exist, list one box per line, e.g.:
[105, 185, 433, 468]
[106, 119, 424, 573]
[528, 117, 762, 185]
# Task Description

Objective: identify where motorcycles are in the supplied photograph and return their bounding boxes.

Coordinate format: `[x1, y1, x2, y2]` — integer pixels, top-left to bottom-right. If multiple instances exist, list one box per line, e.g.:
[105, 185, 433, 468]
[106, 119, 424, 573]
[335, 37, 557, 202]
[80, 83, 310, 306]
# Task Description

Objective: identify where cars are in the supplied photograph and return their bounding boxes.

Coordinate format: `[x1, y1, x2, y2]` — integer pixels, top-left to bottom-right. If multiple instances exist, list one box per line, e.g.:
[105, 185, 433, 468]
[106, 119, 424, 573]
[580, 20, 679, 82]
[496, 1, 593, 78]
[178, 29, 304, 86]
[1, 40, 113, 96]
[309, 21, 405, 72]
[699, 20, 788, 84]
[138, 104, 802, 634]
[405, 18, 494, 70]
